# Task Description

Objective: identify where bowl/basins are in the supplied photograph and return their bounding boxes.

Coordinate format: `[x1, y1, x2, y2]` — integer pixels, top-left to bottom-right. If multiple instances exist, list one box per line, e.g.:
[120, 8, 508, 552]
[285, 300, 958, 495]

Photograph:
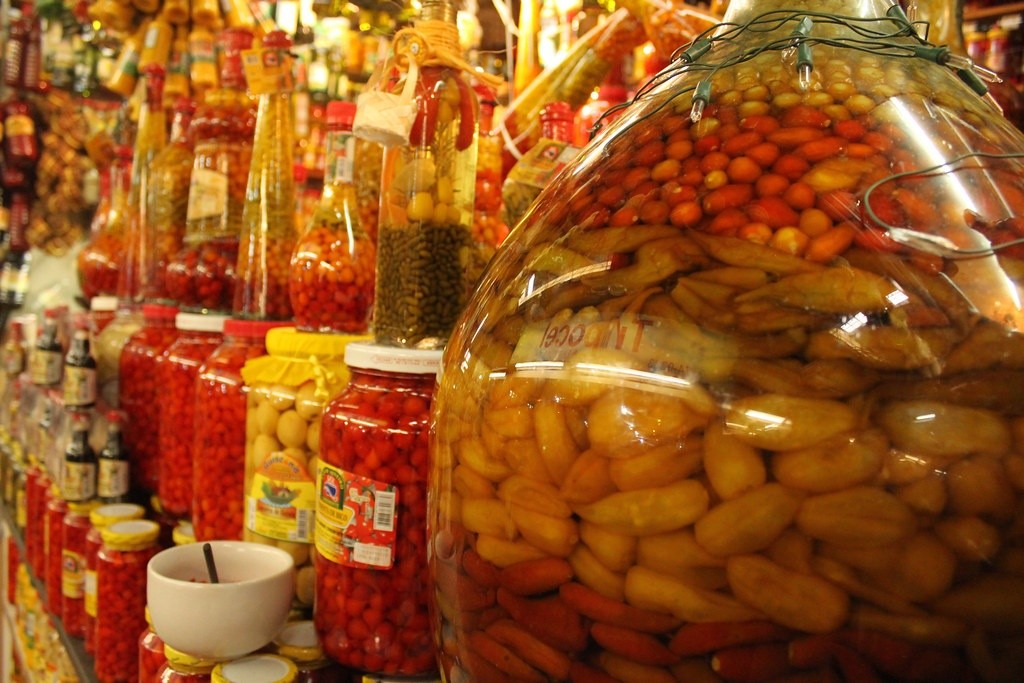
[146, 540, 296, 657]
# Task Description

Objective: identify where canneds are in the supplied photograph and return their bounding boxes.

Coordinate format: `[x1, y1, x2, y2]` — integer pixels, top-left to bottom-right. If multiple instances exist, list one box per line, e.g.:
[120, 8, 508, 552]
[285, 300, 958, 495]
[1, 297, 455, 683]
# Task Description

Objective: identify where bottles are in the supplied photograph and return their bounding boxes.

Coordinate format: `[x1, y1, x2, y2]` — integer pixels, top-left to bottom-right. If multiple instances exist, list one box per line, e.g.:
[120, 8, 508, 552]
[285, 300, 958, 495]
[0, 2, 1024, 683]
